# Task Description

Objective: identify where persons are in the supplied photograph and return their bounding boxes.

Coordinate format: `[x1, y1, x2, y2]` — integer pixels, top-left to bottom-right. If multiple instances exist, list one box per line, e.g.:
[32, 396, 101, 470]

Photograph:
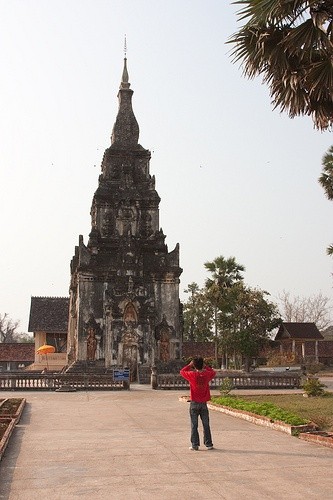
[180, 355, 216, 451]
[18, 362, 25, 368]
[40, 368, 47, 374]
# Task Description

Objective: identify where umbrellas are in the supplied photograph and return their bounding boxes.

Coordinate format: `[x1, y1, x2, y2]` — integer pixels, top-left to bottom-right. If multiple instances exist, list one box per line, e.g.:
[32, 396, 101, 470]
[37, 343, 55, 370]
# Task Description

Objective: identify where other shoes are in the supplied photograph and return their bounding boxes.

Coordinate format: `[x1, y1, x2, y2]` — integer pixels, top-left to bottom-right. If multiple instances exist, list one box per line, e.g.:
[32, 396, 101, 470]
[207, 446, 212, 450]
[189, 447, 198, 450]
